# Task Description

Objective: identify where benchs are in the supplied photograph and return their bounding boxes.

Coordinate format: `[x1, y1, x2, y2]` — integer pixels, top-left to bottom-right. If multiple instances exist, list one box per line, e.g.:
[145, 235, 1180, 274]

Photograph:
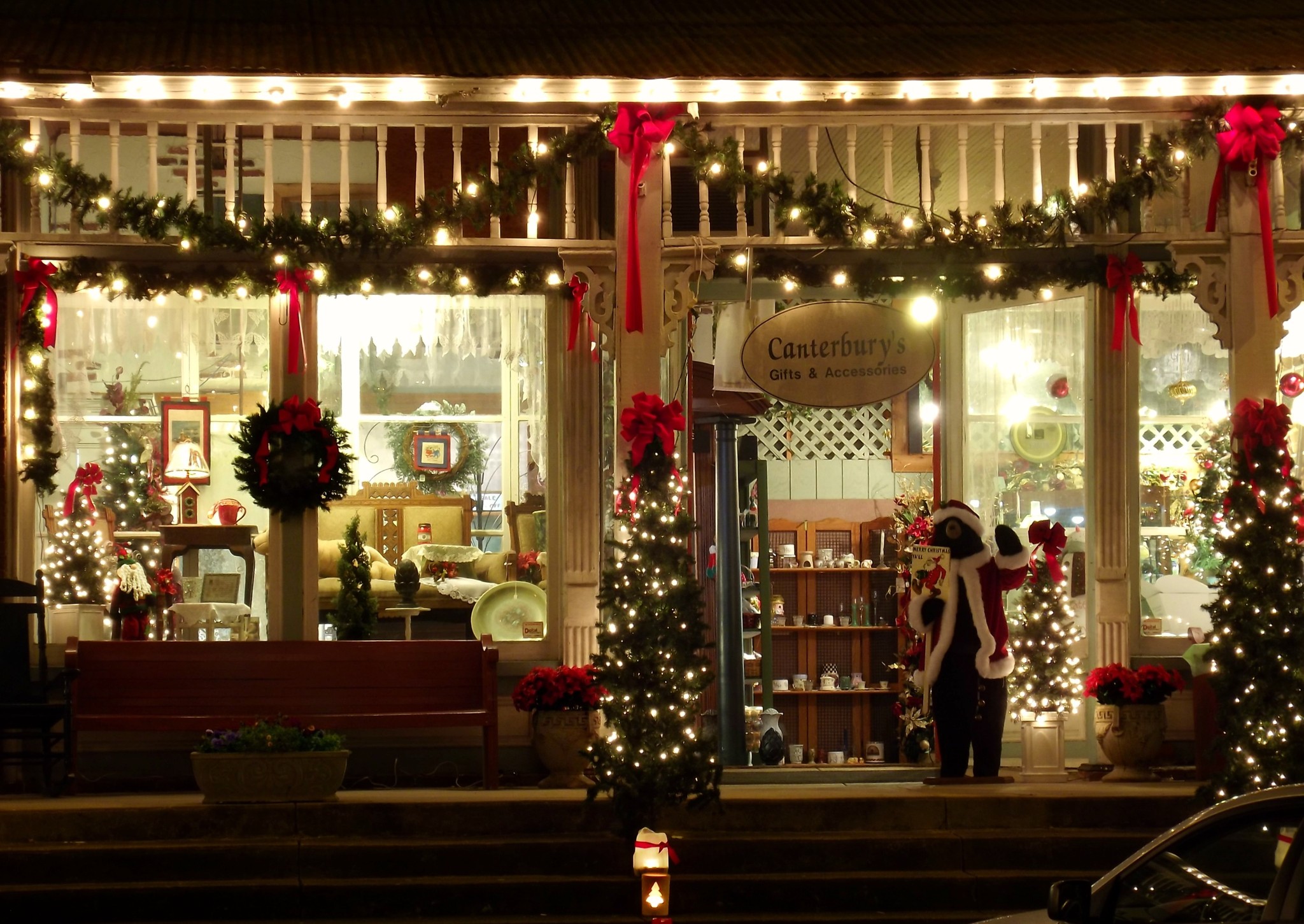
[317, 483, 519, 615]
[64, 635, 498, 789]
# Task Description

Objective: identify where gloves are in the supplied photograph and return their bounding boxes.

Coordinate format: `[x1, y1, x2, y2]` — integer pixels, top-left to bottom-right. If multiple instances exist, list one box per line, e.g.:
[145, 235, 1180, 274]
[920, 598, 945, 626]
[995, 525, 1023, 556]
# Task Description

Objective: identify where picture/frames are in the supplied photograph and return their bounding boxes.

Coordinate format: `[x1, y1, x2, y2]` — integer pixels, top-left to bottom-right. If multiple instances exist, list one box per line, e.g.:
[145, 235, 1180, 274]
[161, 400, 211, 486]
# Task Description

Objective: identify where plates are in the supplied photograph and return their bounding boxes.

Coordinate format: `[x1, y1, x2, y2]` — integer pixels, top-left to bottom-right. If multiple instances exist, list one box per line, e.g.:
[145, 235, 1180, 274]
[1009, 407, 1067, 462]
[875, 687, 891, 690]
[853, 687, 869, 691]
[471, 581, 547, 643]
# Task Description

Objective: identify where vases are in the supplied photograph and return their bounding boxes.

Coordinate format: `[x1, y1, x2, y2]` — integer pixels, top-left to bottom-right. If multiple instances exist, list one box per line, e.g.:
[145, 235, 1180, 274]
[1092, 702, 1166, 781]
[190, 749, 350, 804]
[533, 706, 601, 787]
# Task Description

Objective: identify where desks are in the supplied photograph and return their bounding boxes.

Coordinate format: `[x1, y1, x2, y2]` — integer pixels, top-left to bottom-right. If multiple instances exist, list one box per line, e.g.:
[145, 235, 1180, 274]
[159, 525, 259, 611]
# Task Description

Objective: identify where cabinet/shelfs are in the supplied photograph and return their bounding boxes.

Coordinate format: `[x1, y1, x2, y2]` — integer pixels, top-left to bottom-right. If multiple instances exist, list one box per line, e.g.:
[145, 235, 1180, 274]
[747, 565, 903, 761]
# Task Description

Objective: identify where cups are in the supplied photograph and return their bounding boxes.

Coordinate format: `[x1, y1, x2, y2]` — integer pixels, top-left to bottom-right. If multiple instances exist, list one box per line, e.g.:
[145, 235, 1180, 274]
[218, 504, 246, 525]
[741, 540, 897, 765]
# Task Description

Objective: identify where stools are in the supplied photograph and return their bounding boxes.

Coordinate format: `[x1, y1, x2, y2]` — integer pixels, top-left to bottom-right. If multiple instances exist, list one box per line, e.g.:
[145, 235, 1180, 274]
[169, 602, 249, 641]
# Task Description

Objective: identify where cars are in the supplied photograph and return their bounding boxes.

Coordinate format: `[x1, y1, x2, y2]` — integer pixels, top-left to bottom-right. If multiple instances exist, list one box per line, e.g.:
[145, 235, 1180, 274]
[974, 783, 1304, 924]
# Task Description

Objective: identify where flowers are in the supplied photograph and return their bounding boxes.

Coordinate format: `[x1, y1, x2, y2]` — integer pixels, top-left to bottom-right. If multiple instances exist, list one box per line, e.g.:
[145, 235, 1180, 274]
[618, 394, 685, 464]
[1084, 664, 1184, 703]
[1230, 398, 1293, 449]
[515, 664, 611, 708]
[197, 718, 342, 751]
[889, 480, 933, 763]
[278, 395, 321, 433]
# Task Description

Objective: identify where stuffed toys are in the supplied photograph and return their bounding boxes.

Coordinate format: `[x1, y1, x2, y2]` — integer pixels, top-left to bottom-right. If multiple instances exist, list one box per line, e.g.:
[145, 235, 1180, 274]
[907, 500, 1030, 778]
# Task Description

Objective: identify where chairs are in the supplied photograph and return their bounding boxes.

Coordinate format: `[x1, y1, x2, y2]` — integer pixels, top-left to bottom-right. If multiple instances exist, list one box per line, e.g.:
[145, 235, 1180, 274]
[503, 491, 545, 592]
[0, 570, 65, 794]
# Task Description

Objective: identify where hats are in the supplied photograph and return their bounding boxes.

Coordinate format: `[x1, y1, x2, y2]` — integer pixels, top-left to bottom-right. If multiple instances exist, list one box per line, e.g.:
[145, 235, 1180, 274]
[934, 499, 984, 537]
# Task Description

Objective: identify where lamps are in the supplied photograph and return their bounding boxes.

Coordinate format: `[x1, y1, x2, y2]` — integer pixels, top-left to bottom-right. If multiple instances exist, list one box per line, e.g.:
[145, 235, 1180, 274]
[165, 441, 212, 477]
